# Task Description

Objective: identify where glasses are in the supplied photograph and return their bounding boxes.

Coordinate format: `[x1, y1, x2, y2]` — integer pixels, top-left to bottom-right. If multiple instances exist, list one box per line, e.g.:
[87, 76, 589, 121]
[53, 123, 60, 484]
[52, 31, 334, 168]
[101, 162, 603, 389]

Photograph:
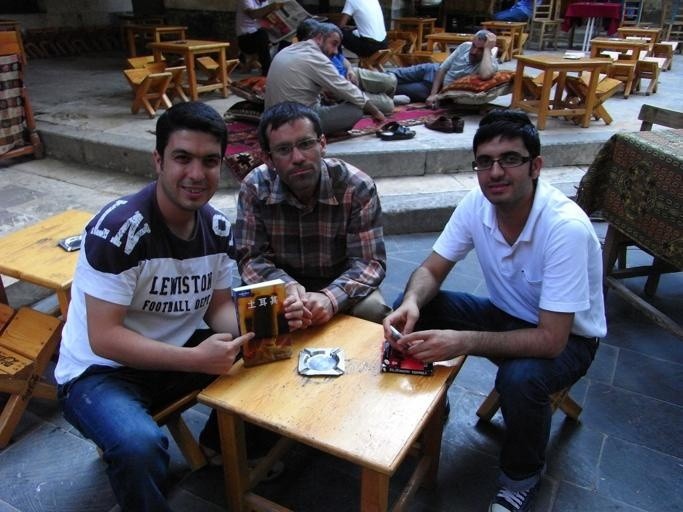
[472, 155, 532, 171]
[268, 138, 320, 155]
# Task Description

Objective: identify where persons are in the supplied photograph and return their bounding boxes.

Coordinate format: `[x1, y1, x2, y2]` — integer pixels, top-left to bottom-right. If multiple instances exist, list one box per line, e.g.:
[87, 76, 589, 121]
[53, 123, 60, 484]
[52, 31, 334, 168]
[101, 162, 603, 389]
[234, 0, 292, 77]
[52, 101, 306, 512]
[380, 105, 610, 512]
[233, 101, 391, 329]
[384, 29, 499, 108]
[336, 0, 387, 59]
[296, 19, 411, 115]
[264, 22, 388, 138]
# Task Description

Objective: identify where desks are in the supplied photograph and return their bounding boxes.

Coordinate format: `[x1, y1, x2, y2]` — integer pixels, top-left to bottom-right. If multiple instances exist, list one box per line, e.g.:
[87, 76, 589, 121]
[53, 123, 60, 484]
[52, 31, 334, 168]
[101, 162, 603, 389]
[0, 208, 97, 325]
[603, 124, 683, 351]
[392, 14, 663, 135]
[196, 311, 467, 511]
[124, 23, 231, 105]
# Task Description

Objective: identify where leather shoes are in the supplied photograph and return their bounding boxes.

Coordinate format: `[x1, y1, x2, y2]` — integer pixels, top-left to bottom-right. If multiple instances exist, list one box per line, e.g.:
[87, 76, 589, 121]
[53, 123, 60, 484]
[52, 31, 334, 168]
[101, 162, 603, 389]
[425, 116, 453, 133]
[452, 115, 464, 132]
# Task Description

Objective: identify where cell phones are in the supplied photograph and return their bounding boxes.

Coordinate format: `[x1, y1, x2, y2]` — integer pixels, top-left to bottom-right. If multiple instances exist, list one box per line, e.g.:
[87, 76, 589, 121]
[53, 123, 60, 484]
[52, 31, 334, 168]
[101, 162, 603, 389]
[388, 324, 410, 347]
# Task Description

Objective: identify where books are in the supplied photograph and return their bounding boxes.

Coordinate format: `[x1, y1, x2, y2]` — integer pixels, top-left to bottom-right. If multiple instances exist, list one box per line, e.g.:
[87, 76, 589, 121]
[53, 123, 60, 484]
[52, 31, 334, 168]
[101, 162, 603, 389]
[231, 278, 294, 368]
[380, 334, 433, 377]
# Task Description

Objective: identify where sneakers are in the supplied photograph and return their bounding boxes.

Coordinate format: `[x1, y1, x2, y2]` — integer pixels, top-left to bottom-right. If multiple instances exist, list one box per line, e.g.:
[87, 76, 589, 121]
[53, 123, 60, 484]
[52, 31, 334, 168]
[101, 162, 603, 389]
[489, 474, 542, 512]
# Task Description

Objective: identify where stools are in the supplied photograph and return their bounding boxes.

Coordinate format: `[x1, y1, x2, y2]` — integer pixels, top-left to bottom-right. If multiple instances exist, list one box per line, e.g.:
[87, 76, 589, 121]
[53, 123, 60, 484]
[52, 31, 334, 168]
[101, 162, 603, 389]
[146, 390, 209, 478]
[123, 69, 175, 121]
[0, 304, 64, 450]
[635, 40, 681, 96]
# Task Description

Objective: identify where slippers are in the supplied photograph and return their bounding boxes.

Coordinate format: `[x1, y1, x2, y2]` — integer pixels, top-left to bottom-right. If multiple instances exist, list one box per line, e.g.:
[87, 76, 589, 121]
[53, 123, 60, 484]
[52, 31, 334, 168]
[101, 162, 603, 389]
[377, 122, 415, 140]
